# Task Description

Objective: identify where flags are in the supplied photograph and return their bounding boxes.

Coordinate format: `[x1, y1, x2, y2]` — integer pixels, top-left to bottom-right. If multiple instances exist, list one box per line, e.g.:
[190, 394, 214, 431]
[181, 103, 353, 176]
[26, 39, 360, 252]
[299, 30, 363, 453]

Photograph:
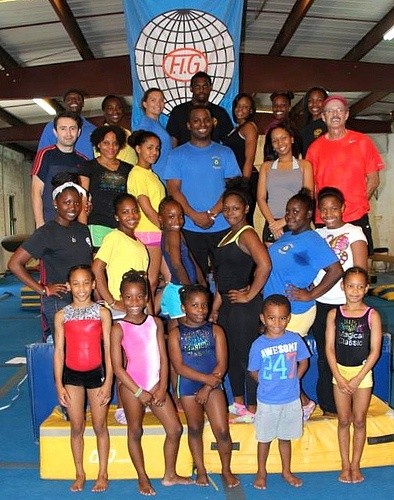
[124, 0, 243, 133]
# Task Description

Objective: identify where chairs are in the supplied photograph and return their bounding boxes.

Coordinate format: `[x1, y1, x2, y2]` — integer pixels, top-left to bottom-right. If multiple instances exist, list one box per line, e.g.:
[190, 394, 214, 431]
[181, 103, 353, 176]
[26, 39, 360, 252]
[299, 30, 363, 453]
[371, 248, 389, 273]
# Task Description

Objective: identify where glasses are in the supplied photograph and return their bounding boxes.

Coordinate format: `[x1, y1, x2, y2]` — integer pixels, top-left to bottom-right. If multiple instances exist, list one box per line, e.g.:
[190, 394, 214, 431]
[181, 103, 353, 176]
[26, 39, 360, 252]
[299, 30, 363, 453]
[323, 107, 347, 115]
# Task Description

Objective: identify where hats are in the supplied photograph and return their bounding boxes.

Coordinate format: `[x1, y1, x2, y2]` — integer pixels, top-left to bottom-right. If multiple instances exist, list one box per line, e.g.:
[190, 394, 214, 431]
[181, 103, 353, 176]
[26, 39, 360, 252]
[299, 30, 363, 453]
[320, 95, 349, 106]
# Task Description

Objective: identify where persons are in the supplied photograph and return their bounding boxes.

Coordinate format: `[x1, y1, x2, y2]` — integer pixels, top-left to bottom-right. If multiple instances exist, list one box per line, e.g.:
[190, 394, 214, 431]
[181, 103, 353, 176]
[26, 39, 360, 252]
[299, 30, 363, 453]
[325, 267, 382, 484]
[6, 173, 94, 420]
[111, 269, 195, 495]
[247, 295, 312, 490]
[224, 93, 259, 230]
[162, 105, 242, 290]
[54, 265, 113, 492]
[262, 187, 344, 422]
[306, 96, 385, 258]
[31, 111, 89, 344]
[35, 73, 369, 424]
[168, 284, 241, 489]
[256, 124, 313, 250]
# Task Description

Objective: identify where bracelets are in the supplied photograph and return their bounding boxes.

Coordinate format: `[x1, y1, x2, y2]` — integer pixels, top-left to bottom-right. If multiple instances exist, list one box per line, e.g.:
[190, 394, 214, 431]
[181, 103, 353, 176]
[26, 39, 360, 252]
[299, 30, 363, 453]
[207, 210, 216, 221]
[134, 387, 142, 398]
